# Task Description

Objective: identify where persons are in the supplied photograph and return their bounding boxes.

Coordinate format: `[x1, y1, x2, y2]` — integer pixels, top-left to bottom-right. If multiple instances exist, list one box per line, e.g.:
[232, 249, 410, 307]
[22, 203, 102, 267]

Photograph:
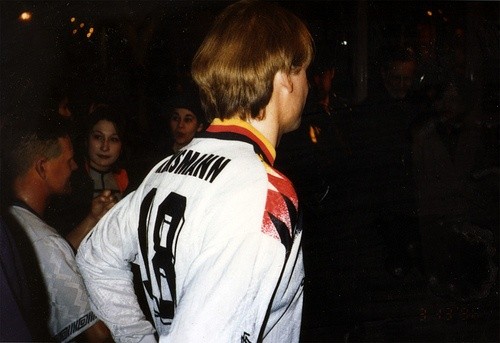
[0, 94, 205, 343]
[273, 47, 464, 276]
[73, 0, 317, 343]
